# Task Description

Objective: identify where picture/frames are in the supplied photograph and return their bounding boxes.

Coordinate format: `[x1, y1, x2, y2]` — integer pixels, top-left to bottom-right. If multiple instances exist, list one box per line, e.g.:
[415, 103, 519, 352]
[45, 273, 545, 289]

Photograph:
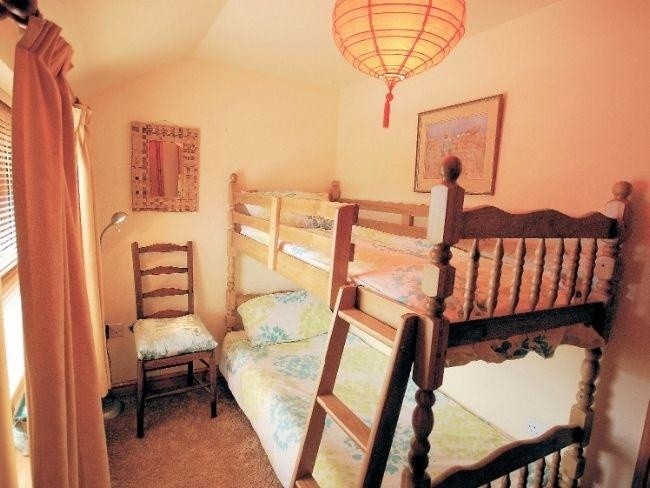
[411, 91, 504, 198]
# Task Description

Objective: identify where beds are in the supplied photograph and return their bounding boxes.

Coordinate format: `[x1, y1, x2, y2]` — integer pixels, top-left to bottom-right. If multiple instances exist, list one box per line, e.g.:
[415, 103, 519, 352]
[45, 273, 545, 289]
[218, 152, 635, 488]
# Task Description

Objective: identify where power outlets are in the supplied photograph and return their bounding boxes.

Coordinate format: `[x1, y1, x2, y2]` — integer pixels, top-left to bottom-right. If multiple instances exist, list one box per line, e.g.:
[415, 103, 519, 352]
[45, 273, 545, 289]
[106, 319, 124, 340]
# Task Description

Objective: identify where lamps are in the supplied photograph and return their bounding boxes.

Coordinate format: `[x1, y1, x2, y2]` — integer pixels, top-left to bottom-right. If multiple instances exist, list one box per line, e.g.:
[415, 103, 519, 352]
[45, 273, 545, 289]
[91, 211, 129, 426]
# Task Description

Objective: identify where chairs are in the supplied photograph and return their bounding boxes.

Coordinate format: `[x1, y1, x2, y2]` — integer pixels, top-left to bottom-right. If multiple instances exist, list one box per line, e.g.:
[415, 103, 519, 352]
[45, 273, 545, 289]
[128, 238, 219, 440]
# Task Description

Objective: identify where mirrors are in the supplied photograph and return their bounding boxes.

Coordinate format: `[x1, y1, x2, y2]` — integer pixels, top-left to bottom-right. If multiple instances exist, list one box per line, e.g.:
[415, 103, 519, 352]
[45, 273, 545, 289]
[128, 118, 203, 212]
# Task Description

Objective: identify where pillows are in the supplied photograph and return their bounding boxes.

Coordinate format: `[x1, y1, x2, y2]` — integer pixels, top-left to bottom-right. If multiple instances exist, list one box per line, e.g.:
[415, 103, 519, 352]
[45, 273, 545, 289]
[238, 187, 336, 232]
[234, 286, 336, 350]
